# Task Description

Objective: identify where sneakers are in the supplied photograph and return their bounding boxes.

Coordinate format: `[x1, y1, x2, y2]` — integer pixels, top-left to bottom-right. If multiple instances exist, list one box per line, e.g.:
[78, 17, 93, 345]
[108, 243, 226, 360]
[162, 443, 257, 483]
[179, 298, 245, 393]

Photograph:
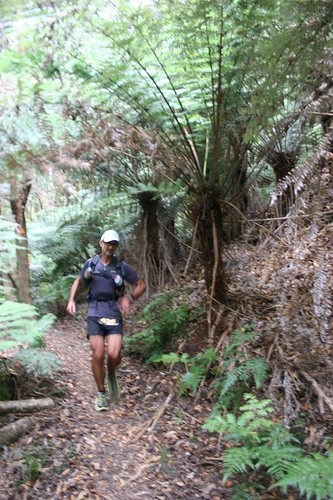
[107, 373, 119, 398]
[95, 395, 109, 412]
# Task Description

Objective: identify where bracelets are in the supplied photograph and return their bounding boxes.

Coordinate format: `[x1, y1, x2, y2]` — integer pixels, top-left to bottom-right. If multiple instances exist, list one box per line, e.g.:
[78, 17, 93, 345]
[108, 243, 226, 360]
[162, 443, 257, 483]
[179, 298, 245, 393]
[128, 293, 135, 302]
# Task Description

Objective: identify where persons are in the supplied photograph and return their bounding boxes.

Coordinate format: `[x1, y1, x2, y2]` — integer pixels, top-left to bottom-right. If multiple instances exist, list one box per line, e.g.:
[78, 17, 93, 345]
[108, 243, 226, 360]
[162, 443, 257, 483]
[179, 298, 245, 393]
[66, 230, 147, 412]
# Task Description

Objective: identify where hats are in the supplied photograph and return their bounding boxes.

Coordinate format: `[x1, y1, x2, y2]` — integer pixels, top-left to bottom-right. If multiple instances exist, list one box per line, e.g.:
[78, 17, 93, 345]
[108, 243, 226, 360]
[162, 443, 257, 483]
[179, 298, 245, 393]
[100, 230, 121, 243]
